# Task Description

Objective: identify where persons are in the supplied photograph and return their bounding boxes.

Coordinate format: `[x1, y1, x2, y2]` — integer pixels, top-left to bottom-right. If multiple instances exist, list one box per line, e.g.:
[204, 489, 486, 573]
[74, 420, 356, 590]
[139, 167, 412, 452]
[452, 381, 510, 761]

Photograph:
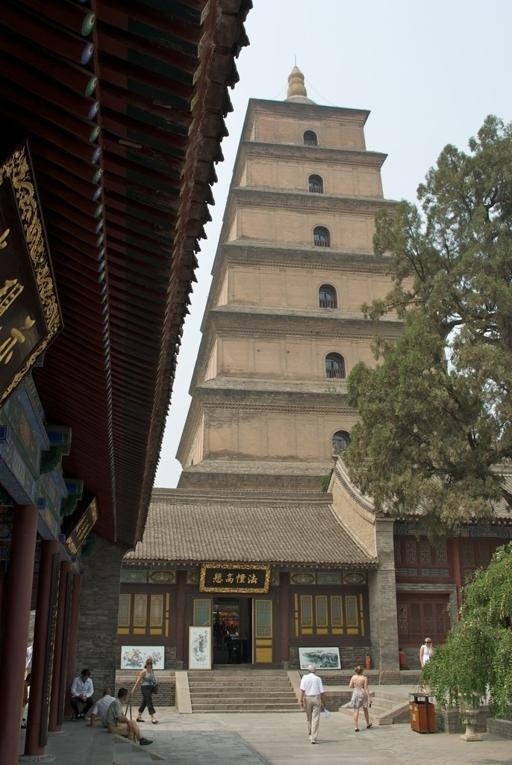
[130, 659, 158, 723]
[420, 637, 435, 668]
[105, 688, 153, 745]
[86, 688, 116, 728]
[349, 665, 373, 732]
[70, 669, 95, 720]
[299, 665, 325, 744]
[20, 637, 32, 728]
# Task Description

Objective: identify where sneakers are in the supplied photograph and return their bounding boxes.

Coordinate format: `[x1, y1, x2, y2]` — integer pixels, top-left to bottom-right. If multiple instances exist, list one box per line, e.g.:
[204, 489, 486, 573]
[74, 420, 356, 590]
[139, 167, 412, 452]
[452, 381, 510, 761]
[152, 720, 158, 724]
[138, 737, 153, 745]
[136, 718, 144, 722]
[354, 723, 372, 732]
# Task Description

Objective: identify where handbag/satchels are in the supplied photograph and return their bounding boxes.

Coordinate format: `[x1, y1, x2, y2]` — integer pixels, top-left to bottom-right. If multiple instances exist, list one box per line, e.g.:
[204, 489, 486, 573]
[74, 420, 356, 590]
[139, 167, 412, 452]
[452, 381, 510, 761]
[152, 684, 160, 694]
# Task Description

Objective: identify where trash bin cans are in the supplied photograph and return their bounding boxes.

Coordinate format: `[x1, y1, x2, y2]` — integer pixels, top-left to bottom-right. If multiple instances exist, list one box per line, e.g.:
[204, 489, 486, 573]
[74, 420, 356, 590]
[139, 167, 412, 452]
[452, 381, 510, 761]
[408, 693, 436, 734]
